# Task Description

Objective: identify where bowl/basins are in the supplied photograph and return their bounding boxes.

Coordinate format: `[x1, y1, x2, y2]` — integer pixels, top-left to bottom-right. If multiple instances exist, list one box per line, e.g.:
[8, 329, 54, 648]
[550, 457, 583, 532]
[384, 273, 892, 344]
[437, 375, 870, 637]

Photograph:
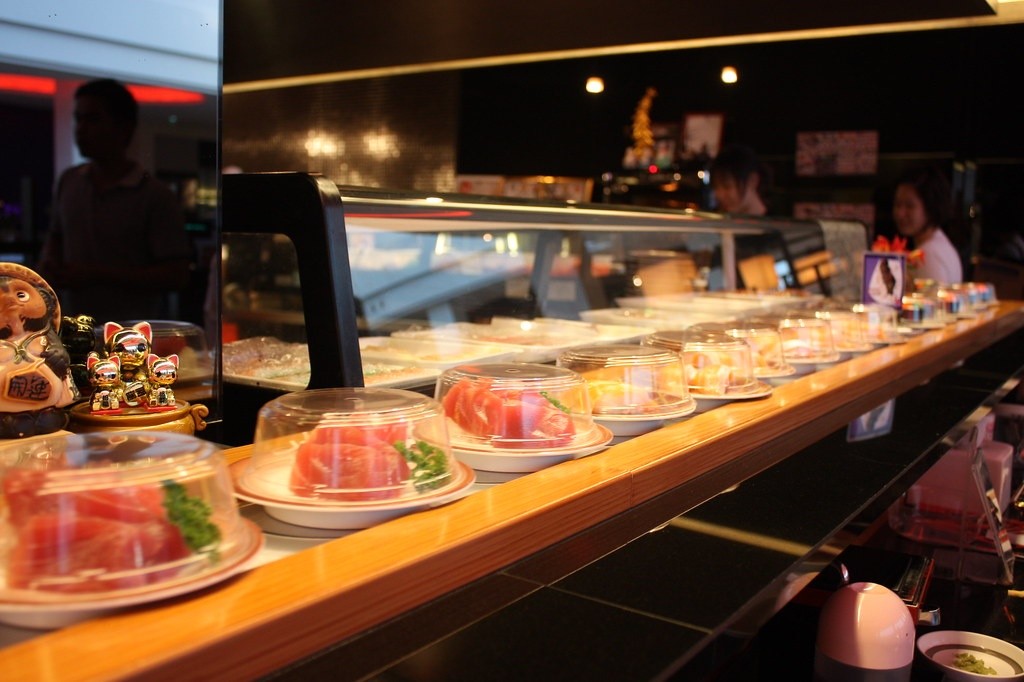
[813, 582, 915, 670]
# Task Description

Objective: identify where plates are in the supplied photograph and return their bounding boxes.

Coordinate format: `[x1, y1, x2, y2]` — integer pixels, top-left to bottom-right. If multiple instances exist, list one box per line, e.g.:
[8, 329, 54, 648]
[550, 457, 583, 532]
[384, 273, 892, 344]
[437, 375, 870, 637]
[625, 372, 773, 412]
[538, 387, 697, 436]
[411, 413, 614, 473]
[0, 517, 264, 629]
[917, 631, 1024, 682]
[170, 364, 217, 389]
[219, 455, 476, 528]
[789, 299, 1006, 383]
[686, 363, 797, 386]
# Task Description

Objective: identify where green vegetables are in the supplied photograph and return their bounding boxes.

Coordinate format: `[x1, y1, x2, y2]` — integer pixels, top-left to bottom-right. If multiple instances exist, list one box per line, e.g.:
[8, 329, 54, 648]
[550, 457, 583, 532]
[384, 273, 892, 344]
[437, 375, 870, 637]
[162, 387, 569, 563]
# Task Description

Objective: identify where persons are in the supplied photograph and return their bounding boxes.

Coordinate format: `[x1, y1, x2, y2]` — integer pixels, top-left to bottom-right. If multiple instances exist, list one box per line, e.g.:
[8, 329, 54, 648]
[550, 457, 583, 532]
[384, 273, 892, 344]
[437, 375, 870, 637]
[32, 78, 185, 316]
[893, 179, 963, 290]
[711, 153, 796, 217]
